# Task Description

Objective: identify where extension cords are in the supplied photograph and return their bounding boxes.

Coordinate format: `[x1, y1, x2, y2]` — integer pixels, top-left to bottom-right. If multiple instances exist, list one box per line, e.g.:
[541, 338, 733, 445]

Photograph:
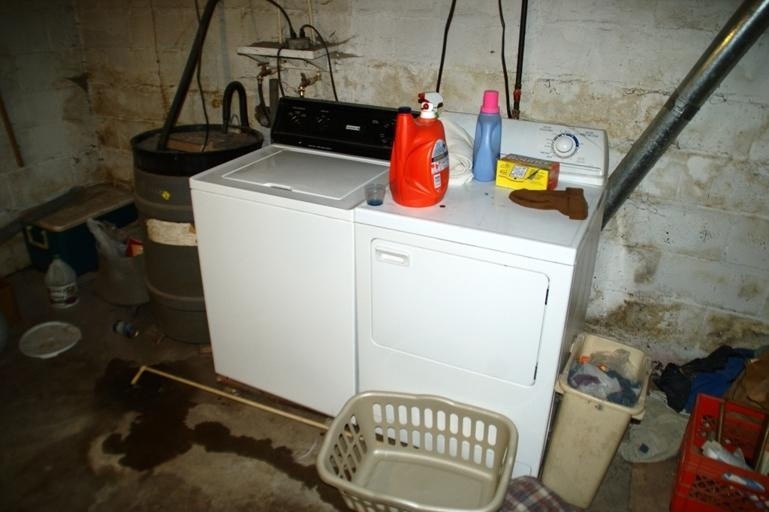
[288, 37, 310, 50]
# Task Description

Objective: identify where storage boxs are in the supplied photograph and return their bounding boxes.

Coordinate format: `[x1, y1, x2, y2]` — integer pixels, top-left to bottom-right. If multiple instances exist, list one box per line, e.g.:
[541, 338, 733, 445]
[23, 186, 136, 275]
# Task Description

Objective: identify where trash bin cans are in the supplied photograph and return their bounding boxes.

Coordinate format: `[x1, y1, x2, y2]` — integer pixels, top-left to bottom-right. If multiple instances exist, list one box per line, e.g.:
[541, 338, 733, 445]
[540, 330, 651, 511]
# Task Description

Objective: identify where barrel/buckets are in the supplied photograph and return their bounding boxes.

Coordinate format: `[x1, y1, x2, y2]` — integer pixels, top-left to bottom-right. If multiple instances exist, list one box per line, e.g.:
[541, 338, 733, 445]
[44, 254, 80, 309]
[130, 124, 264, 345]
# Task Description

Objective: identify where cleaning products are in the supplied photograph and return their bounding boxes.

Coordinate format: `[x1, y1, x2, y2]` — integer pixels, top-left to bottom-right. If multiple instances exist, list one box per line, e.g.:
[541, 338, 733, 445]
[472, 90, 502, 182]
[389, 92, 449, 208]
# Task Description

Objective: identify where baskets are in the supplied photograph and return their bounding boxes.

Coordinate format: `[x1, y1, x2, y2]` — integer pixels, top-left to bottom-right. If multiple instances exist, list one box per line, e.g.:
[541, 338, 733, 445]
[670, 391, 769, 511]
[316, 390, 519, 512]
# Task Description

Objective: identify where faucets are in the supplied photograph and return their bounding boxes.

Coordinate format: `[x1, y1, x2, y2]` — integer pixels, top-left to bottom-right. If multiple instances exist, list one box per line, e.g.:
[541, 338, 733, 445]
[256, 62, 278, 84]
[297, 72, 321, 97]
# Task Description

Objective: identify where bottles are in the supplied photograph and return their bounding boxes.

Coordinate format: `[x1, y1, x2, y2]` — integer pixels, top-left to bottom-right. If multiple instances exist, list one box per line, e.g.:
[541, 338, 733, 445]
[111, 319, 142, 339]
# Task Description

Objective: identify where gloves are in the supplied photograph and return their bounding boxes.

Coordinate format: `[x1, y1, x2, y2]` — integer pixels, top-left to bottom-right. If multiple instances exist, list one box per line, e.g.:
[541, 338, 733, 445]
[509, 186, 589, 221]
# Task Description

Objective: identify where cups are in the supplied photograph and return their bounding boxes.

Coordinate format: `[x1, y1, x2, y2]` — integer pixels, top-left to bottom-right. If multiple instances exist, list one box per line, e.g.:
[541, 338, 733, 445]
[364, 183, 385, 205]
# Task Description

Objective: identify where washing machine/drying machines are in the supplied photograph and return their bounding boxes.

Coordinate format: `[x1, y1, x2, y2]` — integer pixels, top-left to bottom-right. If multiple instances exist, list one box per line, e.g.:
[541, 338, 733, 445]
[355, 108, 610, 484]
[188, 95, 439, 427]
